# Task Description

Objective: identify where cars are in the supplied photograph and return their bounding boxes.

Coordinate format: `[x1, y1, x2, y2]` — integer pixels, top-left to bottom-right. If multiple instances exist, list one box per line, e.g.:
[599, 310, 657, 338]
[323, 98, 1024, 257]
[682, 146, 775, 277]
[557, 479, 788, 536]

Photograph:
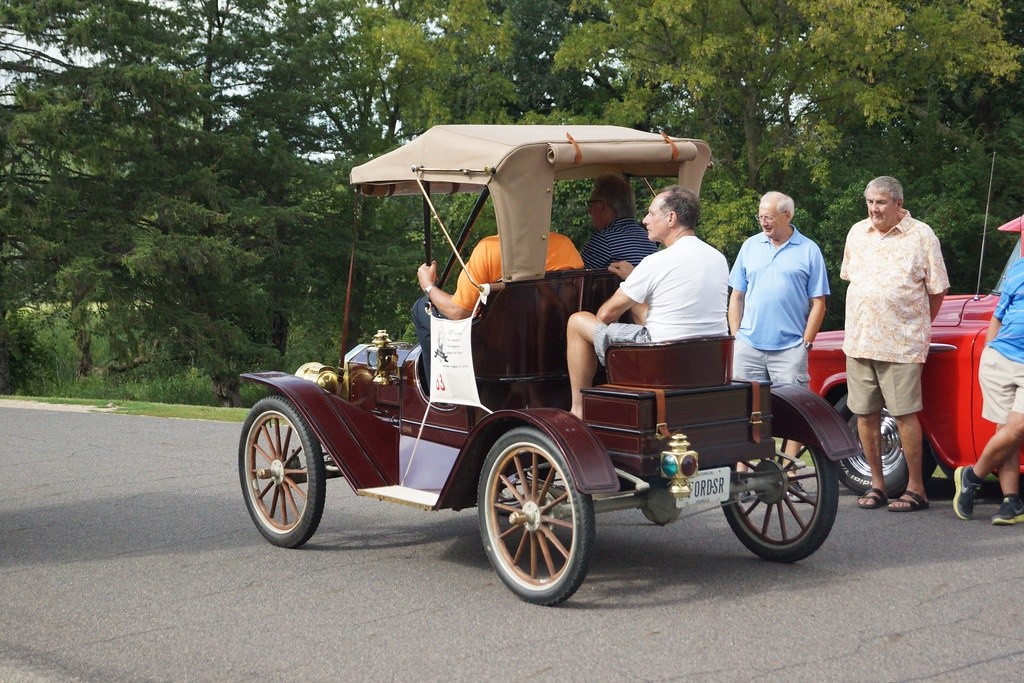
[809, 214, 1023, 498]
[238, 124, 863, 605]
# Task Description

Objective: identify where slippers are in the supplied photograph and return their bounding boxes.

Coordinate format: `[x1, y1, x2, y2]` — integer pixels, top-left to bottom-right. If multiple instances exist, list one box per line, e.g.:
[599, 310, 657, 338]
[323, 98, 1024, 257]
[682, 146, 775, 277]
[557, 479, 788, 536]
[886, 489, 929, 512]
[856, 486, 887, 508]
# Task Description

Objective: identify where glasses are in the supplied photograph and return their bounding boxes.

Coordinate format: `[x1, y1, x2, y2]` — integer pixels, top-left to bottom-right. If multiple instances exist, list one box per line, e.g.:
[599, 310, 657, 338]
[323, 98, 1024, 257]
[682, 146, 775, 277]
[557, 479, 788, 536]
[587, 198, 604, 206]
[755, 210, 786, 222]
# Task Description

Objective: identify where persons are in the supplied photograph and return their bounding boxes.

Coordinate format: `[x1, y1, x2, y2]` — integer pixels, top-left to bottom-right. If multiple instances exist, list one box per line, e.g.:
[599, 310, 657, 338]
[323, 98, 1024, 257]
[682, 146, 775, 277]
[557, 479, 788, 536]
[411, 230, 584, 407]
[839, 176, 950, 512]
[727, 191, 831, 503]
[952, 256, 1024, 526]
[580, 173, 658, 269]
[566, 184, 730, 421]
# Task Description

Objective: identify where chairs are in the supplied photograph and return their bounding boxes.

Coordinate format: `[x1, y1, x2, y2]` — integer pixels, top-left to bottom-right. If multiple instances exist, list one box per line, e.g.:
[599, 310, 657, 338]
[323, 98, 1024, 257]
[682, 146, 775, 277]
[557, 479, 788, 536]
[605, 335, 736, 390]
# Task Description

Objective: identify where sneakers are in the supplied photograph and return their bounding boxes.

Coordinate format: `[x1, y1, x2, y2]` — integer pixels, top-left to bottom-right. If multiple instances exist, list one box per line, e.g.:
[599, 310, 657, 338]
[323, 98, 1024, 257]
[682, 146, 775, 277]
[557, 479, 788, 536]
[991, 495, 1024, 525]
[786, 475, 809, 502]
[952, 465, 983, 521]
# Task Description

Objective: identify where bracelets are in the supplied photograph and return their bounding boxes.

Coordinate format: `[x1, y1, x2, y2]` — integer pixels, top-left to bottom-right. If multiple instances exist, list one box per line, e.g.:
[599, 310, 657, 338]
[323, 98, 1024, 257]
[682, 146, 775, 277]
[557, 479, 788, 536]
[424, 284, 436, 297]
[803, 340, 813, 350]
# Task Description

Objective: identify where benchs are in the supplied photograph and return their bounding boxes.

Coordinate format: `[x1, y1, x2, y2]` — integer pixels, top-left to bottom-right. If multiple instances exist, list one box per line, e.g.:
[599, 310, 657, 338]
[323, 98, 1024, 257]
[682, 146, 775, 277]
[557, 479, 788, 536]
[471, 268, 632, 426]
